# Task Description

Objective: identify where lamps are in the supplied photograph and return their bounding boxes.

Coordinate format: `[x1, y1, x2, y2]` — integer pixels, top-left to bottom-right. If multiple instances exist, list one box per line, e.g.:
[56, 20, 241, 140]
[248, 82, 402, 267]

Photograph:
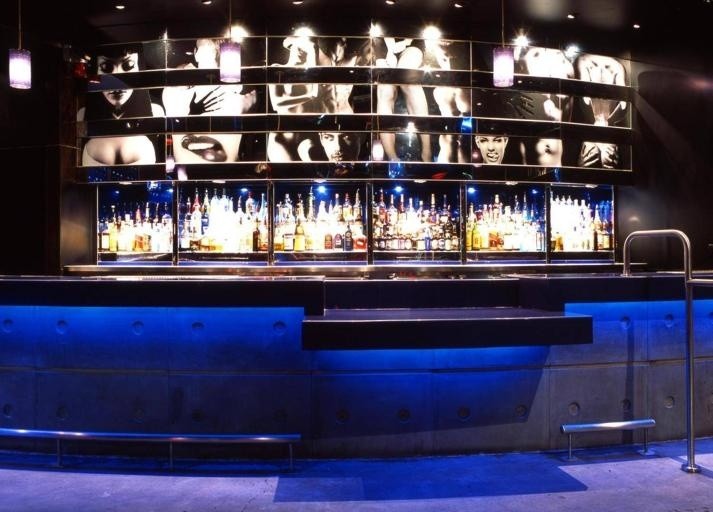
[9, 0, 33, 89]
[220, 0, 241, 82]
[493, 0, 514, 87]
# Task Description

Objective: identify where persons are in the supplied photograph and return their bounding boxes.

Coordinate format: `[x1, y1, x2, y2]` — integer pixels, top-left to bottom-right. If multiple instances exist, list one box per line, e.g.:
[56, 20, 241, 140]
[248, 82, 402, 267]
[77, 38, 629, 169]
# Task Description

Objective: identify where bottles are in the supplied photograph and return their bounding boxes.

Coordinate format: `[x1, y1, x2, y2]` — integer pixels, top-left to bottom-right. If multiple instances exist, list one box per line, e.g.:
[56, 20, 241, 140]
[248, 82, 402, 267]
[97, 193, 611, 254]
